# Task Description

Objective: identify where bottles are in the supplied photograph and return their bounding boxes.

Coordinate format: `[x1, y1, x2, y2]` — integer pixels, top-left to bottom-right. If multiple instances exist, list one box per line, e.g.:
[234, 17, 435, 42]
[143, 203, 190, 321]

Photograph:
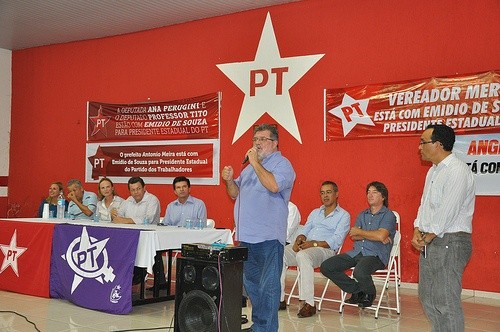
[57, 192, 65, 220]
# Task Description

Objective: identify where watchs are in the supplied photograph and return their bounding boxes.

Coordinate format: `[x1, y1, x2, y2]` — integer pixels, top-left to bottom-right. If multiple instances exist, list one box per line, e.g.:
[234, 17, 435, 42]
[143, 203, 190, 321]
[312, 240, 318, 247]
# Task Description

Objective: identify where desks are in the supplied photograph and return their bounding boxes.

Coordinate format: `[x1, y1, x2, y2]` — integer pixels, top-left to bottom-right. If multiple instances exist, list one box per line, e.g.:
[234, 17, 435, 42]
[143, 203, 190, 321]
[0, 218, 231, 307]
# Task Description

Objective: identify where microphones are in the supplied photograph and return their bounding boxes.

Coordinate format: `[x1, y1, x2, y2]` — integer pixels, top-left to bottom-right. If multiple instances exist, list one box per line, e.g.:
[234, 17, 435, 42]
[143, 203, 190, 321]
[242, 156, 249, 164]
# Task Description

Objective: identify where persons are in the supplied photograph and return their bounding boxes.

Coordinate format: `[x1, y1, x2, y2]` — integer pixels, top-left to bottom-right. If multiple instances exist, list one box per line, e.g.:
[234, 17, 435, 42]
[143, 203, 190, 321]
[319, 182, 397, 308]
[277, 180, 351, 318]
[109, 175, 162, 287]
[144, 176, 209, 291]
[221, 124, 297, 332]
[242, 201, 302, 307]
[409, 124, 477, 331]
[65, 178, 99, 223]
[92, 177, 128, 222]
[36, 182, 70, 220]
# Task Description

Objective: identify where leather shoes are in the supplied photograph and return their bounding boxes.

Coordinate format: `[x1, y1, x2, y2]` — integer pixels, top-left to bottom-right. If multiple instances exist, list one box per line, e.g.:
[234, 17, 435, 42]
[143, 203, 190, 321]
[358, 290, 376, 308]
[344, 291, 362, 304]
[297, 303, 316, 317]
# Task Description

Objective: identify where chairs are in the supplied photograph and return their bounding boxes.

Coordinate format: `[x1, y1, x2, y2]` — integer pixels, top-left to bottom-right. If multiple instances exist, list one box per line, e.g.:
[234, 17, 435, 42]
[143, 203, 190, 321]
[339, 230, 401, 319]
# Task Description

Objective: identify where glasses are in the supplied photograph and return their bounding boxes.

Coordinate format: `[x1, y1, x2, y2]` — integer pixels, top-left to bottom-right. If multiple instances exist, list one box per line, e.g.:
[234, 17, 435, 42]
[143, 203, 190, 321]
[251, 137, 278, 148]
[420, 141, 435, 146]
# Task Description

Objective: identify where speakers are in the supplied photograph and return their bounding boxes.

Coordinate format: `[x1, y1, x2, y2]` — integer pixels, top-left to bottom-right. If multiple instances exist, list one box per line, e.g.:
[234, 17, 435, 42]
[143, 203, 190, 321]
[173, 257, 244, 332]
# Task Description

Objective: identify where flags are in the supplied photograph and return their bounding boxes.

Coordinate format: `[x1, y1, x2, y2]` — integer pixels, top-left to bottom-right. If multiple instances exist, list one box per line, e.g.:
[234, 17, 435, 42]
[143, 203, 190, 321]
[0, 220, 54, 298]
[49, 223, 140, 315]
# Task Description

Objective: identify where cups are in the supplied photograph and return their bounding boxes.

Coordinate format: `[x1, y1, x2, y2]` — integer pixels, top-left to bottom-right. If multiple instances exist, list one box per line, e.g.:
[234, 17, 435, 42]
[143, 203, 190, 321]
[196, 217, 203, 229]
[184, 218, 194, 230]
[42, 204, 49, 220]
[93, 213, 100, 222]
[49, 211, 55, 219]
[66, 211, 71, 220]
[143, 214, 150, 225]
